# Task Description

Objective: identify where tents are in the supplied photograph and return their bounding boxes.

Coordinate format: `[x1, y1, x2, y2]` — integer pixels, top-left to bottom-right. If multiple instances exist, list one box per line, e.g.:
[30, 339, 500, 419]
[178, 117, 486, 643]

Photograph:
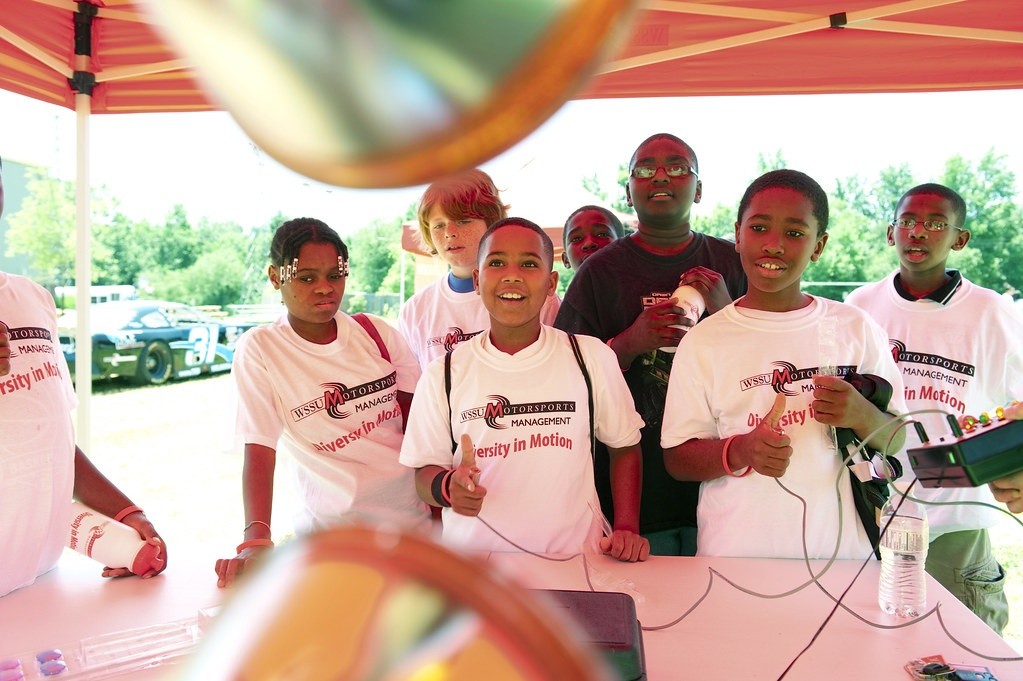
[0, 2, 1022, 461]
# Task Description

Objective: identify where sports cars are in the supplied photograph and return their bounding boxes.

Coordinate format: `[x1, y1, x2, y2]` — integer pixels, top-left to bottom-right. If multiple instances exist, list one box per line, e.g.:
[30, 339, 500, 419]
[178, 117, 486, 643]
[50, 299, 233, 390]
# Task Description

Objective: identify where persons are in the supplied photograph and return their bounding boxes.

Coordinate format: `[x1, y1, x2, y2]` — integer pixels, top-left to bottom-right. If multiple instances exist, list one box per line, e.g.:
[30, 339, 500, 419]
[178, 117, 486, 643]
[0, 157, 166, 599]
[658, 170, 909, 559]
[554, 133, 746, 555]
[843, 182, 1023, 634]
[401, 168, 561, 367]
[562, 206, 623, 271]
[215, 217, 421, 589]
[400, 218, 651, 563]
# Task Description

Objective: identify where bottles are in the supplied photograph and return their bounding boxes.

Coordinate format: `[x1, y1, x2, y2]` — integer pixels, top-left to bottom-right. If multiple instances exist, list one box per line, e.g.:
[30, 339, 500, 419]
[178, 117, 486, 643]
[62, 502, 165, 576]
[877, 480, 930, 619]
[657, 284, 707, 353]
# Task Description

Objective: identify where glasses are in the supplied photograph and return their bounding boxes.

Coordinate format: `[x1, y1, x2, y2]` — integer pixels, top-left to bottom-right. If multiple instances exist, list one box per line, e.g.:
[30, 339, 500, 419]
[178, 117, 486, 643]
[627, 165, 699, 182]
[892, 218, 963, 232]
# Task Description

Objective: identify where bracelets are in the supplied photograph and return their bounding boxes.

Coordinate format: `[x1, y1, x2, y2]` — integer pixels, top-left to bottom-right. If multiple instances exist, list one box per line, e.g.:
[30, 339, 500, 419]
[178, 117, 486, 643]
[244, 520, 271, 531]
[723, 434, 752, 478]
[607, 338, 630, 371]
[115, 506, 143, 520]
[431, 470, 451, 508]
[442, 469, 455, 501]
[236, 539, 274, 553]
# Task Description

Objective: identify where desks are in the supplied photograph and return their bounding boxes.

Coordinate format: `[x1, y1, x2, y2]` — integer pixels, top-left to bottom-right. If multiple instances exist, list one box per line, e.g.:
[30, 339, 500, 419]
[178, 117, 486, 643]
[0, 552, 1023, 681]
